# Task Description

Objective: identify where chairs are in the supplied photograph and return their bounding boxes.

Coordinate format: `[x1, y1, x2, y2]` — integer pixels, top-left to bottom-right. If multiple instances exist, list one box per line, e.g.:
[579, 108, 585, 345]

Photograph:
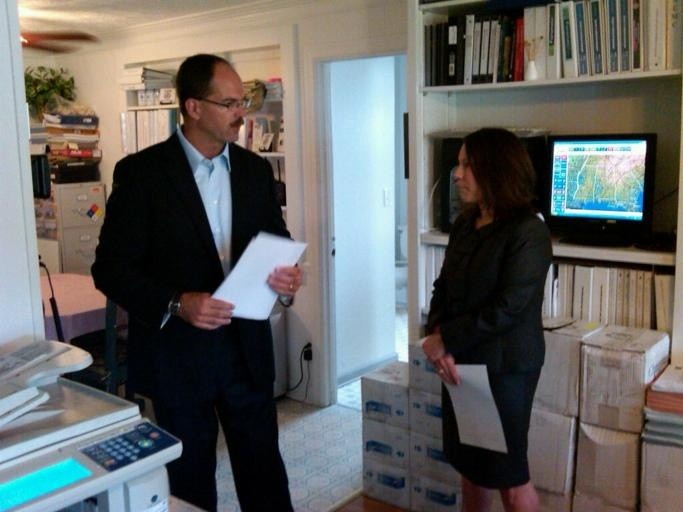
[70, 296, 146, 410]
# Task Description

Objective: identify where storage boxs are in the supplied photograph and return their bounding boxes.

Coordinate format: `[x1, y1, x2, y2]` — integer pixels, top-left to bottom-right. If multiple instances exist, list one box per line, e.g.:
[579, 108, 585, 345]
[515, 315, 601, 422]
[572, 422, 641, 510]
[575, 322, 672, 434]
[638, 440, 680, 510]
[522, 406, 577, 497]
[360, 335, 465, 510]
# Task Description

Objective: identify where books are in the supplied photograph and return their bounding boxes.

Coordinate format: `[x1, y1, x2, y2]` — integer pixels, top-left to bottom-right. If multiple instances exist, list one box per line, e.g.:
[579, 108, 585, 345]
[119, 108, 274, 155]
[424, 0, 683, 87]
[419, 244, 674, 333]
[29, 113, 103, 166]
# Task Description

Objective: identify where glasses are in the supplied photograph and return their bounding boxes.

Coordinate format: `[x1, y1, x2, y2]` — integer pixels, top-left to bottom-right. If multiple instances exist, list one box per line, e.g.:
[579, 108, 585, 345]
[197, 96, 252, 112]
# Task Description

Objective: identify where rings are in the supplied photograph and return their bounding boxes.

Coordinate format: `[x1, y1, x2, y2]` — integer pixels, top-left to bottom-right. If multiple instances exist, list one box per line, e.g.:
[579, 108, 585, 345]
[289, 285, 293, 291]
[438, 369, 444, 374]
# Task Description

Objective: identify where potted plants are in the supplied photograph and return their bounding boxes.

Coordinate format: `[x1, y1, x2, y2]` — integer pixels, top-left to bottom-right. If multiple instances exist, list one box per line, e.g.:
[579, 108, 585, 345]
[25, 65, 76, 120]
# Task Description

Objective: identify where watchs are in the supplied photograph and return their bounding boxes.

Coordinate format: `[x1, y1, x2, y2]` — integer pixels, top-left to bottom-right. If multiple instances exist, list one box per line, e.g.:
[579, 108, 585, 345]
[169, 291, 185, 317]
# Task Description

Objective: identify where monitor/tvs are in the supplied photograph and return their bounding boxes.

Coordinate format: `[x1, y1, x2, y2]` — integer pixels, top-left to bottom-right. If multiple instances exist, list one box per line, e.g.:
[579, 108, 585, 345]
[543, 132, 658, 247]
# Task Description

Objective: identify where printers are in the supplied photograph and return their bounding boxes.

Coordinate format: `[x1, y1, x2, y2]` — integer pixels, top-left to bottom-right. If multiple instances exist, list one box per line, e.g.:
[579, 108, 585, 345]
[0, 333, 185, 512]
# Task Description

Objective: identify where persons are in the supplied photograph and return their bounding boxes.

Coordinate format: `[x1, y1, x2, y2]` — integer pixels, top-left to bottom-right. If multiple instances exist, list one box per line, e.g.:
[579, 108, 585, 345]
[421, 127, 553, 512]
[91, 53, 302, 512]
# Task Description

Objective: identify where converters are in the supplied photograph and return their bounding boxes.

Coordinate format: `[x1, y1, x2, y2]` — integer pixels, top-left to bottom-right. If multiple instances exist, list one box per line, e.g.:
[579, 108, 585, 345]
[302, 347, 311, 360]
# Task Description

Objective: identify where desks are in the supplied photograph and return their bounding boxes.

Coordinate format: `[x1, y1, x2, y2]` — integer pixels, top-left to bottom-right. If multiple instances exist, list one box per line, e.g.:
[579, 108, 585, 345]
[38, 271, 130, 353]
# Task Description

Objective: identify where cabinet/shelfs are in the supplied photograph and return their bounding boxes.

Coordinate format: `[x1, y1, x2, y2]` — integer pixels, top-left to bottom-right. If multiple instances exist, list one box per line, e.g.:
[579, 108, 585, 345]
[408, 0, 682, 370]
